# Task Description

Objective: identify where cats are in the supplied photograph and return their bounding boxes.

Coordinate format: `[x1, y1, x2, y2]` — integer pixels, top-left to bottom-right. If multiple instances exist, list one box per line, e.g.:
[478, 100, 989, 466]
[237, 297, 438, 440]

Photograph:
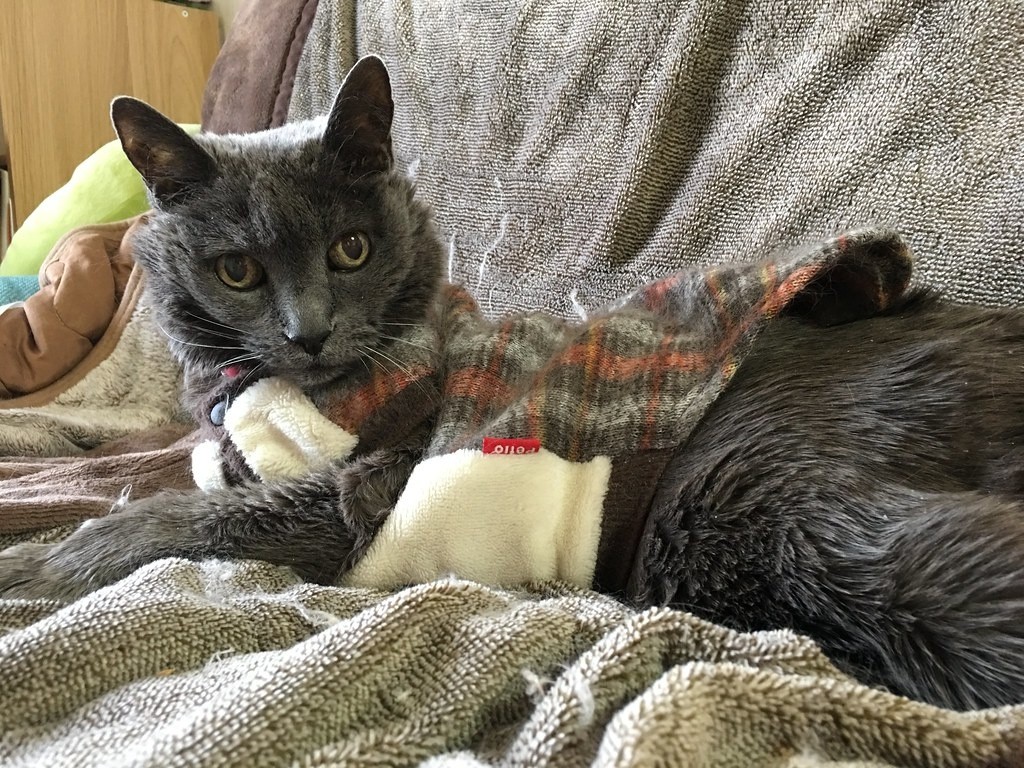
[2, 53, 1023, 707]
